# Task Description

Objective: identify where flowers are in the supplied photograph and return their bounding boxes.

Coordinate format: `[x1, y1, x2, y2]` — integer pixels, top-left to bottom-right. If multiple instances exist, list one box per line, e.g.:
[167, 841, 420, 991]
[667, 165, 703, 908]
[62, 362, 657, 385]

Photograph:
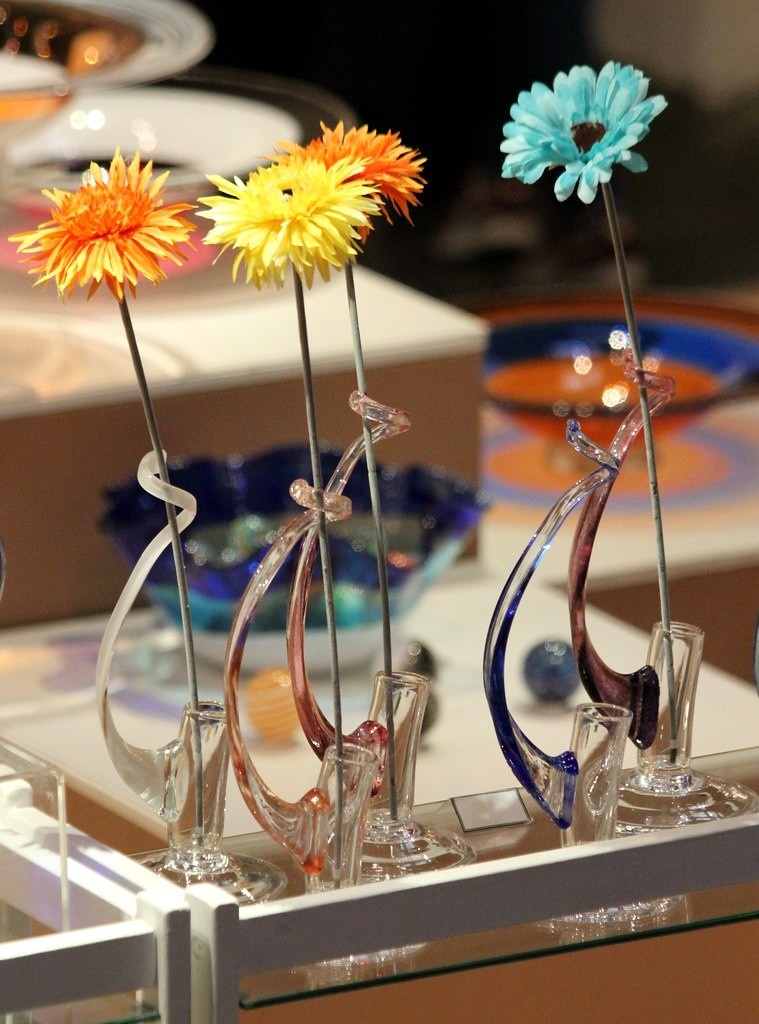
[500, 62, 681, 760]
[8, 118, 428, 755]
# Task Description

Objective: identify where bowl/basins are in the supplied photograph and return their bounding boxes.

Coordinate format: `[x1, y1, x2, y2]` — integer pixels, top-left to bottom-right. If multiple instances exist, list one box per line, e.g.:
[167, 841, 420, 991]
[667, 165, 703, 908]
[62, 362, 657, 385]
[478, 318, 759, 463]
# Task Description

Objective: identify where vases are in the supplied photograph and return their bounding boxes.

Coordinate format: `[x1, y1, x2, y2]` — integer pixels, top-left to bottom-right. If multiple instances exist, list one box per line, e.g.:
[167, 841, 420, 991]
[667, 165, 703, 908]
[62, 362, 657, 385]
[97, 377, 759, 903]
